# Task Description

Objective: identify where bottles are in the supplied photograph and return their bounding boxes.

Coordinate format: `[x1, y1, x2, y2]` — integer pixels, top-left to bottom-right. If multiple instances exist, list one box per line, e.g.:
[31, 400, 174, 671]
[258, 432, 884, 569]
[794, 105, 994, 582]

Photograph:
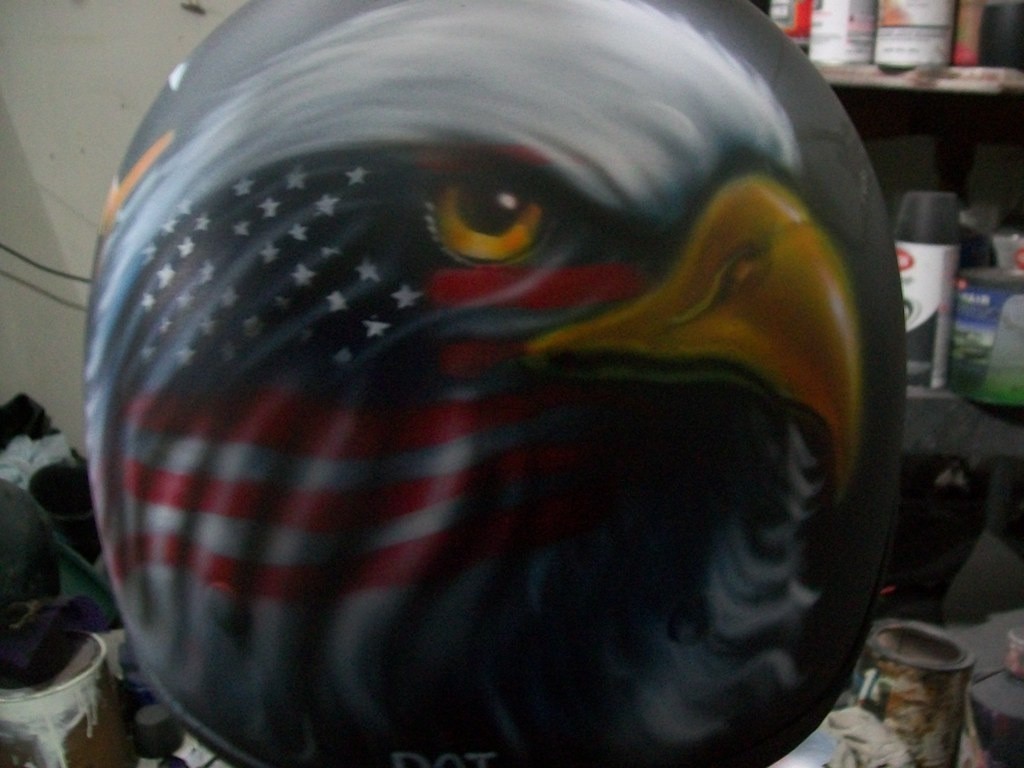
[965, 627, 1024, 768]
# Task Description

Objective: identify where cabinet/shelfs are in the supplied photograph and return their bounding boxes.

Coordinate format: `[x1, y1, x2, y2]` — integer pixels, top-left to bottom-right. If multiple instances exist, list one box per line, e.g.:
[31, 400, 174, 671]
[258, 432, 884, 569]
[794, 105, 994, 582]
[817, 65, 1024, 459]
